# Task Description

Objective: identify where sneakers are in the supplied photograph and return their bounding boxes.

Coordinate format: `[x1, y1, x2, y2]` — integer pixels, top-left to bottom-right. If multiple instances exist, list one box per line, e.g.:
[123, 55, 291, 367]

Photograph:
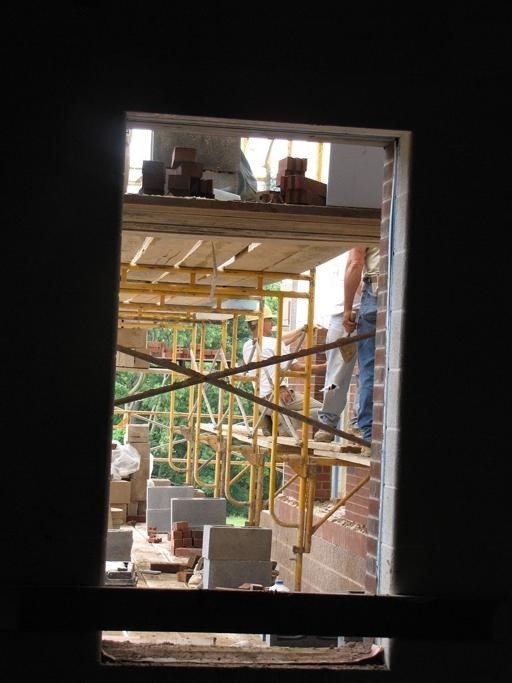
[314, 429, 334, 442]
[260, 416, 272, 436]
[271, 413, 291, 437]
[361, 446, 371, 456]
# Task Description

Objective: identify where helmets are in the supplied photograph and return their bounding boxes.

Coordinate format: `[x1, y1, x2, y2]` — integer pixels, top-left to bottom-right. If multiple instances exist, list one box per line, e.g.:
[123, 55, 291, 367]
[245, 304, 273, 322]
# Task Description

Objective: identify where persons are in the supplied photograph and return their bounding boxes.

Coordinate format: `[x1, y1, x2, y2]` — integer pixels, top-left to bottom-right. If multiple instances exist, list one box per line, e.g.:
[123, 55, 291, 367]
[342, 241, 380, 458]
[313, 251, 363, 442]
[245, 305, 327, 436]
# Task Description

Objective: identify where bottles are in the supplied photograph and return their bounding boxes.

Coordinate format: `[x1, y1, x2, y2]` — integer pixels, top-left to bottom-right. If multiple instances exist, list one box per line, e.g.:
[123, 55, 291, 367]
[269, 580, 289, 592]
